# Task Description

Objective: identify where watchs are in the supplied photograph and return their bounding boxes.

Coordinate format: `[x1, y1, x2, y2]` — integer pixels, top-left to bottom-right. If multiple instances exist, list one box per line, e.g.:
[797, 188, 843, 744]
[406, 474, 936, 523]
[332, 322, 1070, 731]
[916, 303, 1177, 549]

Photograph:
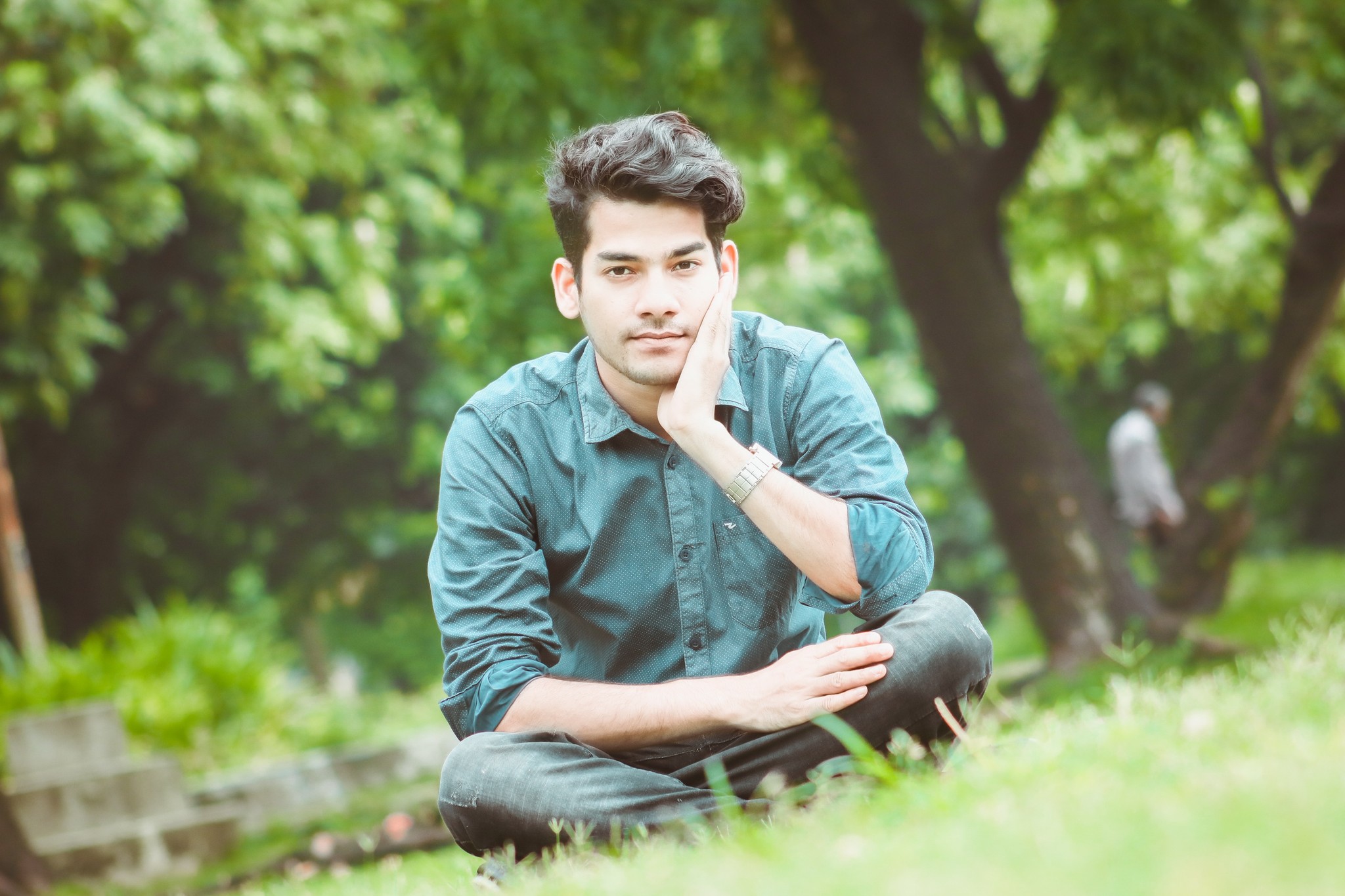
[722, 442, 784, 510]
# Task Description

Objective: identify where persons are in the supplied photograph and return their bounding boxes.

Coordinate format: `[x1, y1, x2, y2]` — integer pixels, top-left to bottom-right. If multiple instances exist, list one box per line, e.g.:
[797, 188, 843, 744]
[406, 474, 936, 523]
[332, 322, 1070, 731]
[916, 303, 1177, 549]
[427, 109, 993, 891]
[1107, 379, 1187, 543]
